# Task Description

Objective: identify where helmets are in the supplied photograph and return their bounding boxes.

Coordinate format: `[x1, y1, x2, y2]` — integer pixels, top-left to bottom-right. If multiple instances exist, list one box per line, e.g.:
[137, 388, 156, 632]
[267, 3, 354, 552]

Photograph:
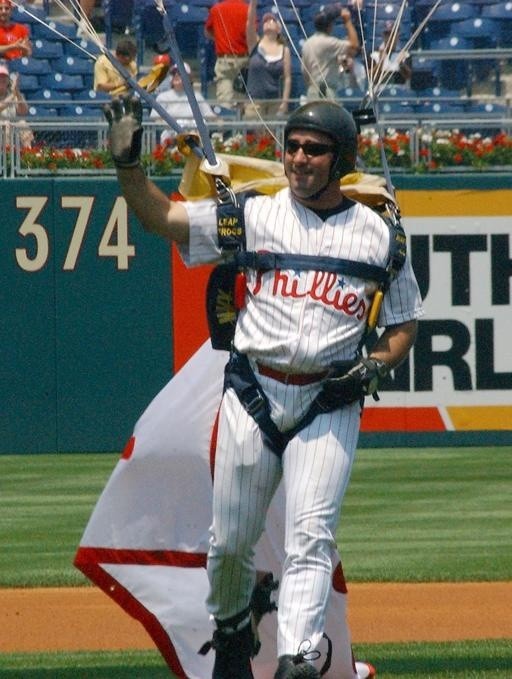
[284, 100, 357, 182]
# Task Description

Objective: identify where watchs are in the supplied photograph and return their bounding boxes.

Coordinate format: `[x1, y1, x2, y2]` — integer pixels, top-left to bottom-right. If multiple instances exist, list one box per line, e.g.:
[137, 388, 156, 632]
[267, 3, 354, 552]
[367, 357, 388, 390]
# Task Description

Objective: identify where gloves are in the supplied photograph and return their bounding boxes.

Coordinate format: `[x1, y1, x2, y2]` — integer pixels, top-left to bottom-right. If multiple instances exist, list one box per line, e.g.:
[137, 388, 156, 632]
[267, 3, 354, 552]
[103, 93, 144, 167]
[323, 357, 388, 405]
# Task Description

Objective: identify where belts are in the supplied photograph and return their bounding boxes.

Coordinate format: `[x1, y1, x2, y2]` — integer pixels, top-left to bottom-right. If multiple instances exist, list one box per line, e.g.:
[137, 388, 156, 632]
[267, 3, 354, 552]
[258, 363, 328, 386]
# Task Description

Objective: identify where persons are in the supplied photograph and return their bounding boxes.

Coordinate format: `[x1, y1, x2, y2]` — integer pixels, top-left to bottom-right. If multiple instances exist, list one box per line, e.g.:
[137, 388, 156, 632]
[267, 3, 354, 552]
[0, 0, 34, 149]
[103, 94, 427, 679]
[488, 59, 512, 106]
[77, 0, 413, 142]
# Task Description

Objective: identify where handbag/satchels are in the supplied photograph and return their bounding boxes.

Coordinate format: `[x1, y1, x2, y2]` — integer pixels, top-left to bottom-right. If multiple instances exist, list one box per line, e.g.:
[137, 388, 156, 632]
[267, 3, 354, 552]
[233, 67, 249, 94]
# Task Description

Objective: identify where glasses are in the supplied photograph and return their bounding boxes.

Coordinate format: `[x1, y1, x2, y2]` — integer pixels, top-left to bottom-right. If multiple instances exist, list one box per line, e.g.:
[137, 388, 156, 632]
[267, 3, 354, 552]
[287, 139, 334, 156]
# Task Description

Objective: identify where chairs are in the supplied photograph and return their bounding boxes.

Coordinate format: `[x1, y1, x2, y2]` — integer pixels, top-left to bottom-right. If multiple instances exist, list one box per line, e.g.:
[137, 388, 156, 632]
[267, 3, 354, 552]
[1, 2, 512, 117]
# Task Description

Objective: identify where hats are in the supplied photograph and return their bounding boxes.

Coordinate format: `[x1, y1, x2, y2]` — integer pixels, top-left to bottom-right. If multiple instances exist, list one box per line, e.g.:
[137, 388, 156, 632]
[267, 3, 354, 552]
[169, 62, 191, 74]
[262, 12, 282, 30]
[382, 20, 400, 34]
[312, 2, 342, 26]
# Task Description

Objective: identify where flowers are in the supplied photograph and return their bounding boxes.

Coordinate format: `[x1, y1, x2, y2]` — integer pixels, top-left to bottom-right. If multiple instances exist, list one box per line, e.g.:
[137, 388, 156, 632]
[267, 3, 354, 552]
[0, 127, 512, 172]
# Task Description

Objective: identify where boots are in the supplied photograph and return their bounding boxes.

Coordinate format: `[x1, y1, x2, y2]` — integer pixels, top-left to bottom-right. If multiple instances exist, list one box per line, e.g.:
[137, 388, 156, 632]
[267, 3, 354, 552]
[205, 605, 261, 679]
[274, 653, 320, 679]
